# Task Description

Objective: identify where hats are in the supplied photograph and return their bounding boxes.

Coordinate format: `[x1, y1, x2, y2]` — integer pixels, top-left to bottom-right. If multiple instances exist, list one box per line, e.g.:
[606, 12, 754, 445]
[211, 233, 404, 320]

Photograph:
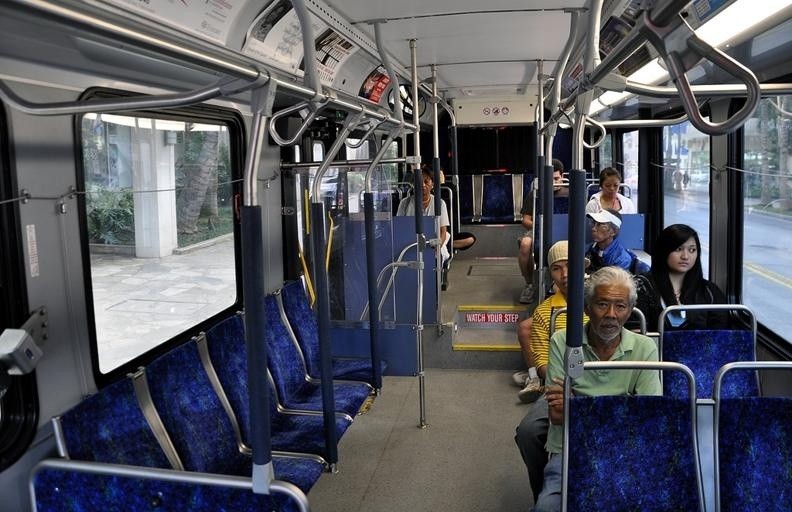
[586, 208, 621, 227]
[547, 239, 591, 268]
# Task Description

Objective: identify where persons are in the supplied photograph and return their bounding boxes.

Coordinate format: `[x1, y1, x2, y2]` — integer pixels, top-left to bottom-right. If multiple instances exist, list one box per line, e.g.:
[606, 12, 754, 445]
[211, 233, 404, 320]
[520, 157, 569, 303]
[628, 224, 732, 332]
[513, 208, 632, 403]
[584, 166, 636, 214]
[515, 242, 593, 511]
[531, 266, 662, 512]
[396, 159, 476, 287]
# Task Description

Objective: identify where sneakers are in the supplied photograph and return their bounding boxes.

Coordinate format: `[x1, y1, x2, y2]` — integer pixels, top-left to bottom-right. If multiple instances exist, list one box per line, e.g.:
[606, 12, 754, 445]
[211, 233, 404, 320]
[518, 284, 537, 304]
[514, 370, 543, 403]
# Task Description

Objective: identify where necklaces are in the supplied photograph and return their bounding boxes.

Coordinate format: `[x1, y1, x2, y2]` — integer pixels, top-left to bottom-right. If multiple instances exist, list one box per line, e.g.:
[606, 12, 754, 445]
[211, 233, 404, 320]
[607, 200, 616, 209]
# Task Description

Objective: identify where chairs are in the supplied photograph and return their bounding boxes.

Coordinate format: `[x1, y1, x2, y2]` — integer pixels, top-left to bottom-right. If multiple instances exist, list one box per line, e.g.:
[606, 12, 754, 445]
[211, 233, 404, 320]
[471, 168, 521, 225]
